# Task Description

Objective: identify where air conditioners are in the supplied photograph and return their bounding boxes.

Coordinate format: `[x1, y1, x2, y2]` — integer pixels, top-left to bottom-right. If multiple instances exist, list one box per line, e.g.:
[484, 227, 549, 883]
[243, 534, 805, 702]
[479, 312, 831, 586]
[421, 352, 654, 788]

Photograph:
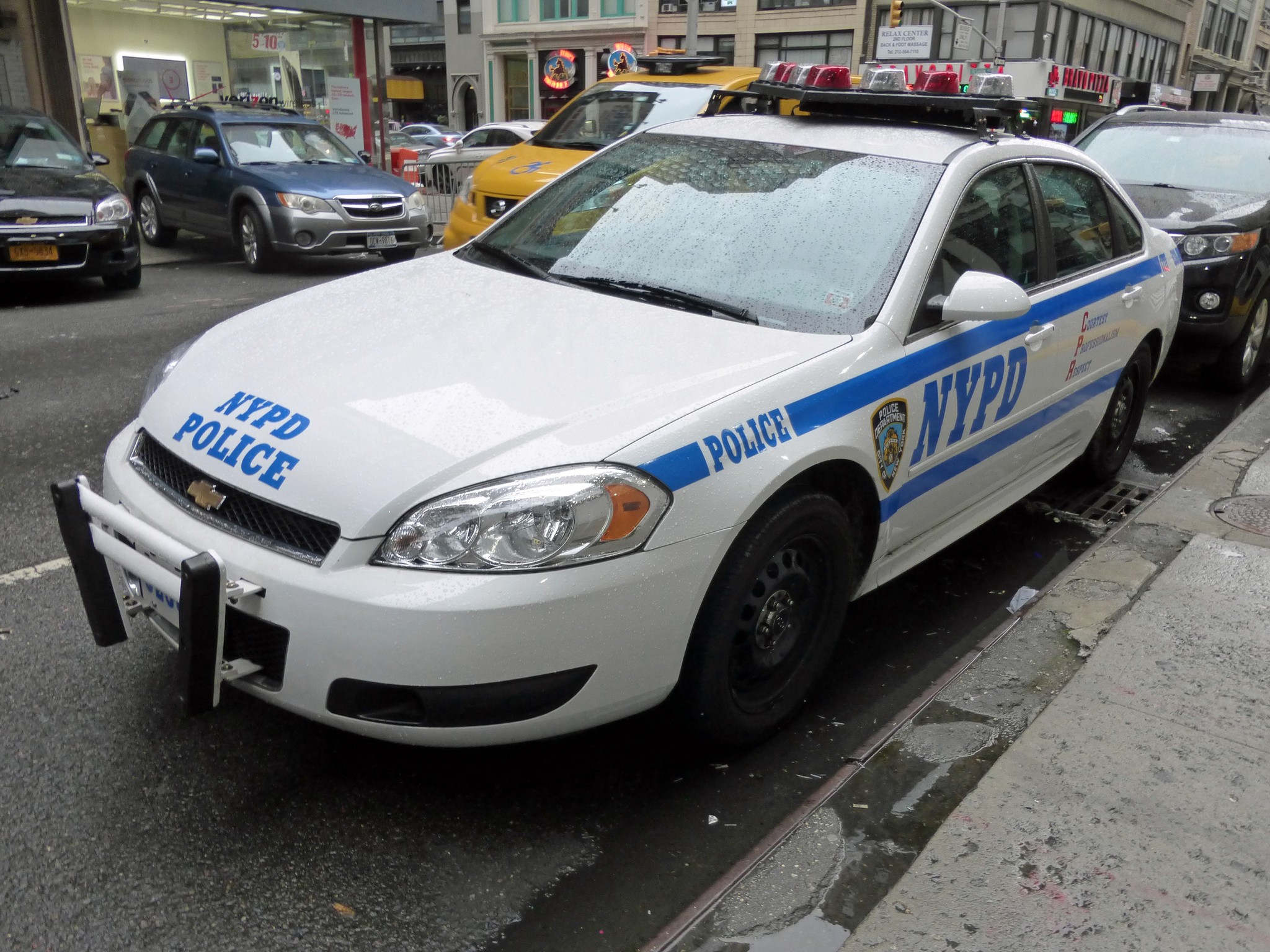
[1247, 75, 1260, 85]
[661, 3, 674, 13]
[1256, 78, 1266, 86]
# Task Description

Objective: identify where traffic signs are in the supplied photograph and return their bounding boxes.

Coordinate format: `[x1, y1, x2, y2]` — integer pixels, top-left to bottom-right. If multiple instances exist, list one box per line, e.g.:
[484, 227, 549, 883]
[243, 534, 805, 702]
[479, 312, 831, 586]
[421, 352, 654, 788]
[953, 23, 972, 50]
[993, 56, 1006, 67]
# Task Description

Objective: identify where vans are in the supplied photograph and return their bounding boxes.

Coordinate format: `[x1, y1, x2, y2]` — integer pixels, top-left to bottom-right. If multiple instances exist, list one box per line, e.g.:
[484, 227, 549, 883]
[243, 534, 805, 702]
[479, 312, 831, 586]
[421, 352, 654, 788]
[441, 49, 865, 253]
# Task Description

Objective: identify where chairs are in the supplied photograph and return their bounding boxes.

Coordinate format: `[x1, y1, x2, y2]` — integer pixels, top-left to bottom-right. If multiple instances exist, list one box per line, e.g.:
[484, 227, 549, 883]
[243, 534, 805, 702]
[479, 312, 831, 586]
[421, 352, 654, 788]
[169, 128, 205, 159]
[203, 135, 221, 157]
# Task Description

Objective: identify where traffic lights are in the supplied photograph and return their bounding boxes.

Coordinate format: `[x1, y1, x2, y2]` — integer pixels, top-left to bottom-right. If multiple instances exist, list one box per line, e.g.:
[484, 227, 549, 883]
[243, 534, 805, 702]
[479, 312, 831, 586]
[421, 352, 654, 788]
[889, 0, 904, 29]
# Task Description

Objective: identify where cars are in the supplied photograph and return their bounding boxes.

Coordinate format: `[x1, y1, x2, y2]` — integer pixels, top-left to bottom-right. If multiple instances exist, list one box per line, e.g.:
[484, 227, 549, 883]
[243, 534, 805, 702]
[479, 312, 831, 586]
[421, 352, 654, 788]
[48, 62, 1184, 781]
[373, 130, 436, 180]
[0, 105, 143, 291]
[396, 123, 465, 150]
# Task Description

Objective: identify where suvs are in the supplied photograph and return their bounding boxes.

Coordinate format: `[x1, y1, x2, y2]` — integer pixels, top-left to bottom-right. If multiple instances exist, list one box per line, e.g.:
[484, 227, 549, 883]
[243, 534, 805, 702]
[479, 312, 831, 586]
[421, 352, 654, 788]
[1066, 102, 1270, 392]
[426, 119, 550, 194]
[123, 97, 435, 273]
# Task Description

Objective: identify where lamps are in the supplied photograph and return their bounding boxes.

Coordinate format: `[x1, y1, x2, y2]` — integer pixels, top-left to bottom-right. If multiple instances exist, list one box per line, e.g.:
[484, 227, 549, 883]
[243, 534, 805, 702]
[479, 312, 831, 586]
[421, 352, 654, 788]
[415, 65, 421, 70]
[436, 64, 442, 69]
[426, 64, 432, 70]
